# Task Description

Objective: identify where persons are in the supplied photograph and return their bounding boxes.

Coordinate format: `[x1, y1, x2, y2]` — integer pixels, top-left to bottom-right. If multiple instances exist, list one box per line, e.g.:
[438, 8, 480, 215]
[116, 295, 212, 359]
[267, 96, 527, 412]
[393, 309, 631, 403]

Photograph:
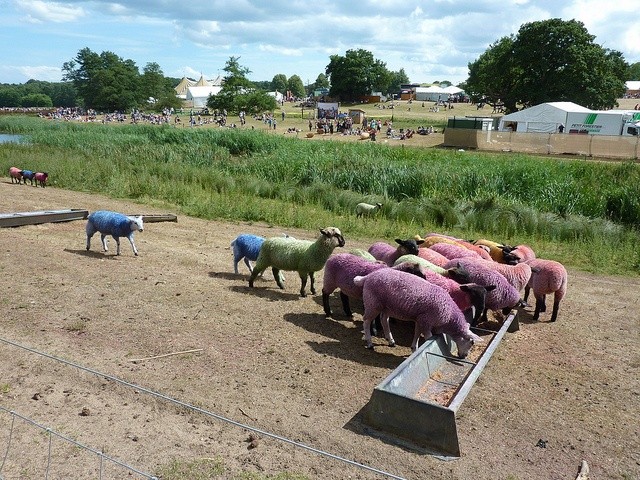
[37, 106, 96, 121]
[624, 92, 640, 99]
[289, 95, 303, 102]
[214, 109, 228, 127]
[379, 120, 381, 128]
[273, 119, 276, 129]
[190, 109, 193, 115]
[507, 122, 514, 131]
[558, 123, 565, 133]
[171, 107, 175, 113]
[244, 117, 245, 124]
[408, 108, 411, 112]
[180, 107, 183, 114]
[254, 112, 273, 128]
[374, 102, 400, 109]
[282, 109, 285, 120]
[638, 107, 640, 110]
[363, 117, 367, 130]
[282, 100, 284, 106]
[447, 94, 470, 103]
[443, 101, 448, 109]
[429, 107, 439, 112]
[422, 103, 425, 107]
[388, 120, 392, 135]
[369, 119, 374, 128]
[408, 98, 413, 104]
[417, 125, 433, 134]
[514, 123, 516, 131]
[175, 115, 180, 124]
[352, 98, 369, 104]
[439, 94, 442, 99]
[197, 110, 200, 115]
[241, 118, 244, 125]
[101, 107, 171, 124]
[634, 104, 638, 110]
[316, 107, 353, 135]
[309, 119, 312, 131]
[406, 129, 413, 137]
[376, 120, 380, 133]
[189, 115, 213, 126]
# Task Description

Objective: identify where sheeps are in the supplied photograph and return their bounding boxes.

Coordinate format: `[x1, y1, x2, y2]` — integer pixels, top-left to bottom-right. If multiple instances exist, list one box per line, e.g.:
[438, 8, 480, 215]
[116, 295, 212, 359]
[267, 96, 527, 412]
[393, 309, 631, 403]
[419, 247, 450, 267]
[369, 238, 424, 266]
[422, 267, 497, 323]
[32, 171, 49, 188]
[456, 239, 493, 261]
[85, 210, 144, 256]
[424, 233, 490, 254]
[524, 258, 567, 322]
[468, 239, 517, 262]
[349, 248, 386, 265]
[248, 227, 346, 297]
[393, 254, 471, 284]
[22, 170, 36, 185]
[355, 202, 384, 221]
[442, 258, 532, 326]
[508, 243, 536, 264]
[429, 243, 482, 260]
[230, 234, 290, 282]
[321, 253, 427, 336]
[353, 267, 484, 359]
[465, 255, 532, 293]
[414, 234, 467, 250]
[8, 167, 24, 184]
